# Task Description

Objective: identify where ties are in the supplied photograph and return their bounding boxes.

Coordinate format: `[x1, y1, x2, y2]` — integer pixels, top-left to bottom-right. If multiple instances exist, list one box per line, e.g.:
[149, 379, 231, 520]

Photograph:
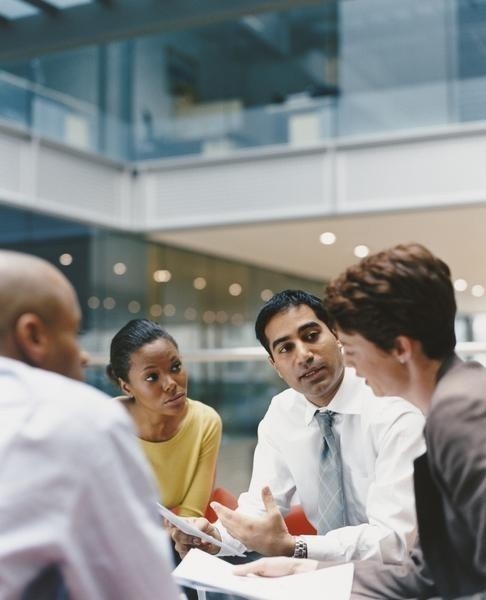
[314, 411, 349, 536]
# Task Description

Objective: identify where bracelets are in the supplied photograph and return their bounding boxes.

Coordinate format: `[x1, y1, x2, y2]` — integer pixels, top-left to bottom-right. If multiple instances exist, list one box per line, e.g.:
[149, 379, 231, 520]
[293, 535, 307, 559]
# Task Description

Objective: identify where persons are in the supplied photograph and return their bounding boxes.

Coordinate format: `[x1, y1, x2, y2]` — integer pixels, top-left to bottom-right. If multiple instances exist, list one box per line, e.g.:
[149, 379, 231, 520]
[168, 289, 428, 563]
[0, 248, 188, 599]
[230, 243, 486, 600]
[102, 318, 223, 540]
